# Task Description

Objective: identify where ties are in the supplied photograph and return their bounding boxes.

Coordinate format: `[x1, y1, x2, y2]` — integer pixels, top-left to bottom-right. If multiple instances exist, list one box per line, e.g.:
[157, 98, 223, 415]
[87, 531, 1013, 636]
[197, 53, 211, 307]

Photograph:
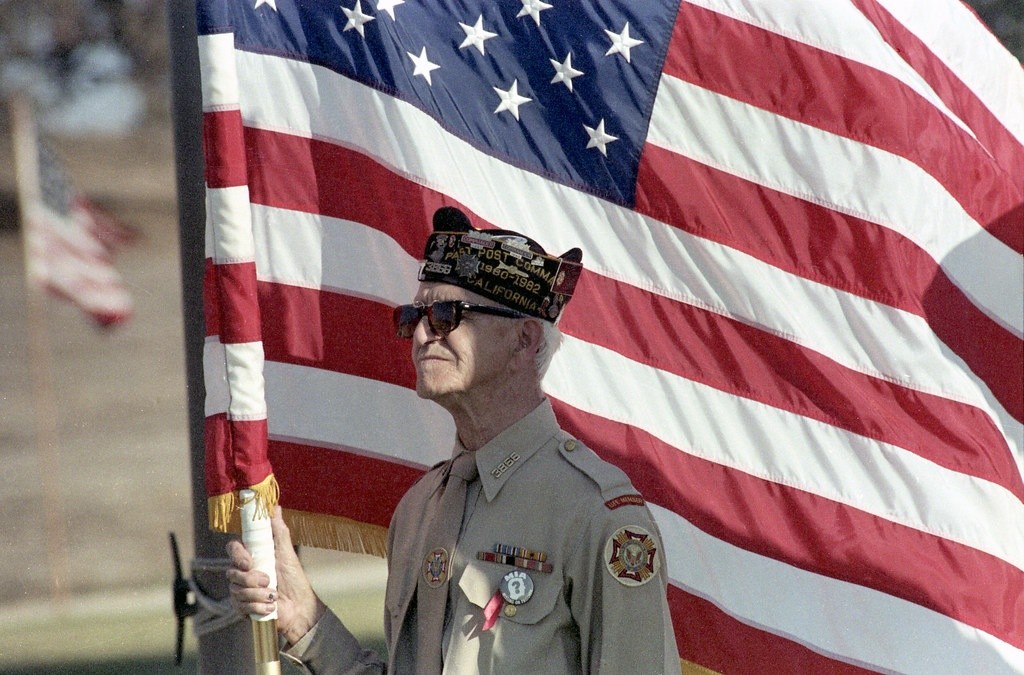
[414, 451, 480, 675]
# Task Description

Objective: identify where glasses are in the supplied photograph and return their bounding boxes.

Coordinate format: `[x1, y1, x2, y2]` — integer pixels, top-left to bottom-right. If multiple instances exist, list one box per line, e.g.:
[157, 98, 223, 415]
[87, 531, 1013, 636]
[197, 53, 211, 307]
[392, 301, 526, 338]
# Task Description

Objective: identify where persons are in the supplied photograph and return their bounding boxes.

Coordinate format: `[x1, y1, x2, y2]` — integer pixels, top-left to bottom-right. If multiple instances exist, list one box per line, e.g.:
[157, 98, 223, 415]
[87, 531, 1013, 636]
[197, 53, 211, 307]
[225, 206, 682, 675]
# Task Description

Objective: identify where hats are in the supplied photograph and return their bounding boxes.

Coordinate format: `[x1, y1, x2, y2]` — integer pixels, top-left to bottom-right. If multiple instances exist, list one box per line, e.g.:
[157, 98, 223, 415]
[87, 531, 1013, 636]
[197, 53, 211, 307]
[417, 204, 585, 324]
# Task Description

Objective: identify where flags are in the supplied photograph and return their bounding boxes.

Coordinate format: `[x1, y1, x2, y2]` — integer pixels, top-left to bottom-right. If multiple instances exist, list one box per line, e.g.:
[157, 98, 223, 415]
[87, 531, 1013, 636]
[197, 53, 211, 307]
[26, 130, 141, 332]
[195, 0, 1024, 675]
